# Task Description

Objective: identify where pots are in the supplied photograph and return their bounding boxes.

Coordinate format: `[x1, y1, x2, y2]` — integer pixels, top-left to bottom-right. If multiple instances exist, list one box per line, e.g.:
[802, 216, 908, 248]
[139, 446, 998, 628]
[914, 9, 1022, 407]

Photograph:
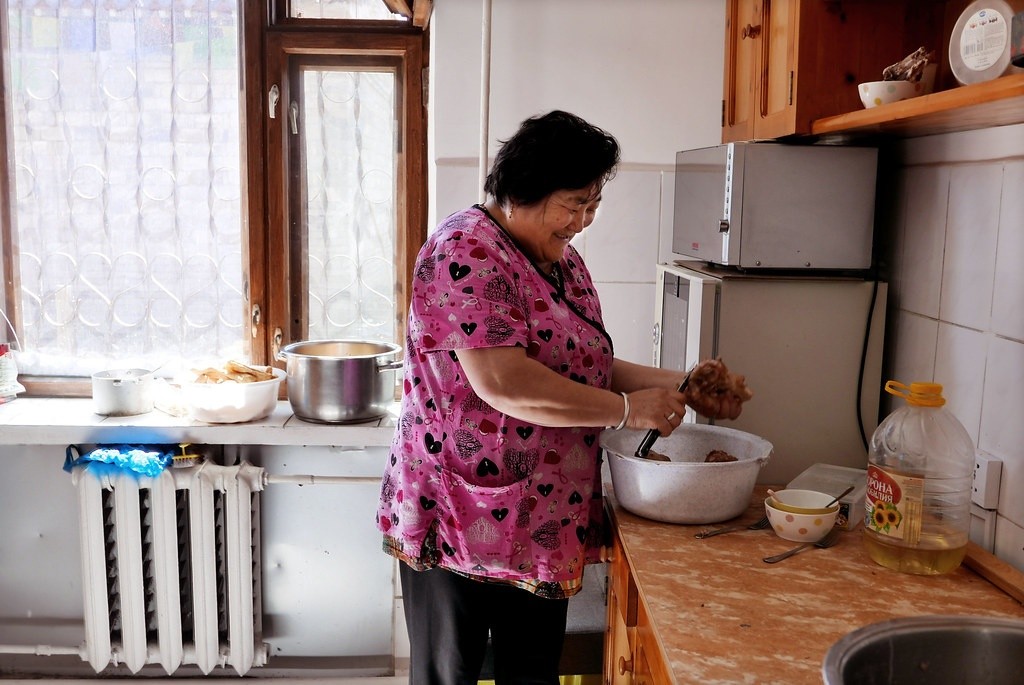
[279, 340, 404, 422]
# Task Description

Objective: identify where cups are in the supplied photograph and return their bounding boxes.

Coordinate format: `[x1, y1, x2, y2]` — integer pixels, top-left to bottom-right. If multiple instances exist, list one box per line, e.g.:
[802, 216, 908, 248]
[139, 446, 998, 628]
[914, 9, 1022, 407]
[916, 64, 938, 95]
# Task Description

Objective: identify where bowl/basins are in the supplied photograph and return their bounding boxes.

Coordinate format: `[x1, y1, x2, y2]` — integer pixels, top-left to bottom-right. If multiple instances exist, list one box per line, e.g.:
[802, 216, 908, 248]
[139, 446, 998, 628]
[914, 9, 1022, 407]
[765, 497, 840, 542]
[92, 370, 152, 416]
[182, 366, 286, 423]
[822, 614, 1024, 685]
[600, 423, 773, 524]
[771, 490, 839, 514]
[858, 80, 925, 109]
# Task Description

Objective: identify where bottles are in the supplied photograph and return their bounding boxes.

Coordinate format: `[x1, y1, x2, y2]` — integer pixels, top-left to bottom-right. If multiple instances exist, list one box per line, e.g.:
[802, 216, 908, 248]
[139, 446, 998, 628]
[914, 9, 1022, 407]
[865, 381, 974, 576]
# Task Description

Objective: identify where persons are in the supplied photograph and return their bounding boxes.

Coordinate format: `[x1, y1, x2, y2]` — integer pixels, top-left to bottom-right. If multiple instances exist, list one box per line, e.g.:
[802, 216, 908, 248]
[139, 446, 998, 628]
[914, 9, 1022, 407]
[374, 109, 743, 685]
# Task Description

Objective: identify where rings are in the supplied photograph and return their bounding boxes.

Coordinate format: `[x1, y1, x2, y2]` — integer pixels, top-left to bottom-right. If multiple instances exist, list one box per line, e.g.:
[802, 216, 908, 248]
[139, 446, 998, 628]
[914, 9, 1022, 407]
[667, 411, 675, 421]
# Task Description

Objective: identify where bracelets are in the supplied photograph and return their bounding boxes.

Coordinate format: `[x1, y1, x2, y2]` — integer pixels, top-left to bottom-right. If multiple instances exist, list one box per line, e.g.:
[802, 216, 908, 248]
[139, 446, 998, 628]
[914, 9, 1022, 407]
[606, 392, 629, 431]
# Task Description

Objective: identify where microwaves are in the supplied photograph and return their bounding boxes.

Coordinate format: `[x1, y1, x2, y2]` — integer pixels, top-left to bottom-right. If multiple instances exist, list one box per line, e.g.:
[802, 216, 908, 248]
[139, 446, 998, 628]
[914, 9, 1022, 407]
[673, 143, 879, 271]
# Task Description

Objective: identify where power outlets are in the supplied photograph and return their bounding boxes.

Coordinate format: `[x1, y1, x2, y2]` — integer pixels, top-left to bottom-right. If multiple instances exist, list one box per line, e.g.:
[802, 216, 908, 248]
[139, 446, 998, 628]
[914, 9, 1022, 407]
[971, 449, 1002, 509]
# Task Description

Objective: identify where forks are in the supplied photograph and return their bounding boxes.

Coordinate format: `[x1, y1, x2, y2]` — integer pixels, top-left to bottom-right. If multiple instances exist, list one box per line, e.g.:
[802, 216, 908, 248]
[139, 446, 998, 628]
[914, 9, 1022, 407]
[694, 516, 770, 539]
[763, 533, 839, 563]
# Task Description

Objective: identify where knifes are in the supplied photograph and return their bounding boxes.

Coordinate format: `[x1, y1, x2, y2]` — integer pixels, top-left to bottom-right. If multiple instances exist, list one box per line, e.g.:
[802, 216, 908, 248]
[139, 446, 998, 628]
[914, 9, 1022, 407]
[636, 363, 696, 458]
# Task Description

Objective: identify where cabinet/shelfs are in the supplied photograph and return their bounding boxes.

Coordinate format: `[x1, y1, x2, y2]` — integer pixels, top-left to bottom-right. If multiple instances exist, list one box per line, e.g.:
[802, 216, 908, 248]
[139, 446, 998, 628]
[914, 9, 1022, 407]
[721, 0, 904, 143]
[603, 490, 656, 685]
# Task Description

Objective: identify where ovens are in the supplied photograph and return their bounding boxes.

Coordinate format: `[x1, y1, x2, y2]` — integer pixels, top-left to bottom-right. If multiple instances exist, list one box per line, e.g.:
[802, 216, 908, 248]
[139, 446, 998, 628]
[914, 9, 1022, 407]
[652, 260, 888, 484]
